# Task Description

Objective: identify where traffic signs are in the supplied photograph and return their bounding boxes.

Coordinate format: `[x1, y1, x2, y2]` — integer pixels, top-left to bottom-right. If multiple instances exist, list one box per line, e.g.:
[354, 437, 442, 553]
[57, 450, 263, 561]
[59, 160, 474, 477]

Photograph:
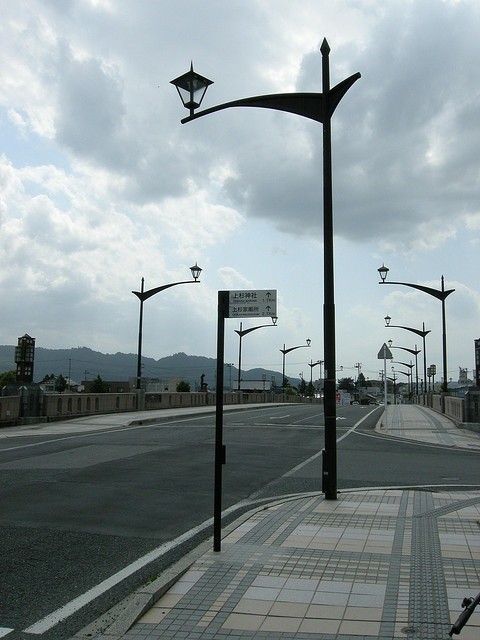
[228, 290, 277, 318]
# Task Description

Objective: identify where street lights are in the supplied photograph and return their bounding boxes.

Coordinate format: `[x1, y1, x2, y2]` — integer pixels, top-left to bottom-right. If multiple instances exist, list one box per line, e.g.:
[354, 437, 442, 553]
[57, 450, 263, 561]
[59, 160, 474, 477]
[384, 313, 432, 412]
[131, 262, 203, 403]
[386, 339, 423, 404]
[169, 35, 362, 503]
[380, 370, 397, 404]
[308, 360, 324, 394]
[234, 317, 278, 390]
[390, 357, 415, 404]
[391, 366, 411, 404]
[336, 366, 343, 372]
[279, 338, 311, 392]
[377, 262, 456, 414]
